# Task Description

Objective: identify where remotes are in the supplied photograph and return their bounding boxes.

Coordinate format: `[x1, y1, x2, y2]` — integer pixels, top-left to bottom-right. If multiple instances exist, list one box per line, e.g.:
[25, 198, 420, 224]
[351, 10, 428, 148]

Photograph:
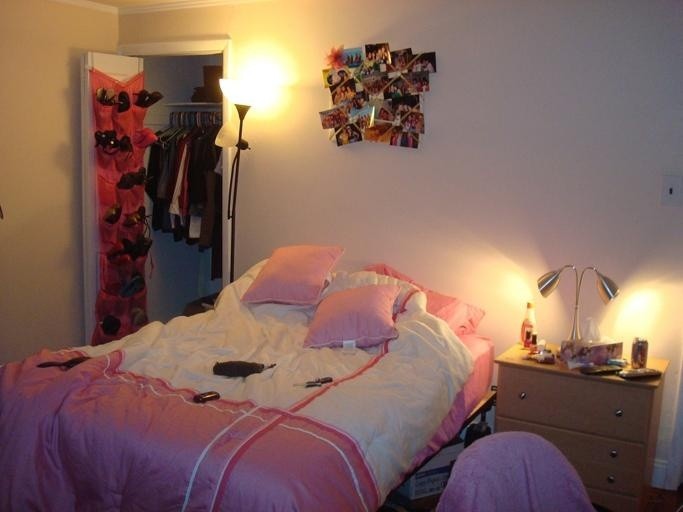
[619, 367, 663, 380]
[579, 364, 622, 375]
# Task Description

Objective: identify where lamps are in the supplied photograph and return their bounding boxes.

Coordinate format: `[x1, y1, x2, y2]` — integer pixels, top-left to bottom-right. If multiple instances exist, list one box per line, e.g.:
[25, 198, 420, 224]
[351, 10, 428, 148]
[536, 264, 621, 344]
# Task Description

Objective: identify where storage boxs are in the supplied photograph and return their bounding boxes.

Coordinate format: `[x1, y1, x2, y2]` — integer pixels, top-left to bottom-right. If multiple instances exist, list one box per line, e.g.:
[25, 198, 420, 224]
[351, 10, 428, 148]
[395, 437, 465, 500]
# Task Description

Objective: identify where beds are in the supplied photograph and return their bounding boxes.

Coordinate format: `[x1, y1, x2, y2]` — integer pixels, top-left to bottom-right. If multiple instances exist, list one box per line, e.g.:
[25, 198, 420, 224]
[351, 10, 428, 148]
[0, 257, 495, 510]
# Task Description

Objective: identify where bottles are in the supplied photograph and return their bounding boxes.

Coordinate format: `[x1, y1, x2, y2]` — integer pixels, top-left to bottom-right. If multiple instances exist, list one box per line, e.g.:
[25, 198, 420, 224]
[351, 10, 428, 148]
[520, 302, 536, 342]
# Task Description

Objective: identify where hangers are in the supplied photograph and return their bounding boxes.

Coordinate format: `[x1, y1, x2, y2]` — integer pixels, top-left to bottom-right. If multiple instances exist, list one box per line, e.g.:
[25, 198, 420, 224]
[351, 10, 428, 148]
[149, 110, 222, 150]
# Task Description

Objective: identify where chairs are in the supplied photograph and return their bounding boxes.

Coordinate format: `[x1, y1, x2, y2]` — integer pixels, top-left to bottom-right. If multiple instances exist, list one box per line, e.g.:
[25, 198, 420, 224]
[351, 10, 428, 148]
[434, 431, 597, 512]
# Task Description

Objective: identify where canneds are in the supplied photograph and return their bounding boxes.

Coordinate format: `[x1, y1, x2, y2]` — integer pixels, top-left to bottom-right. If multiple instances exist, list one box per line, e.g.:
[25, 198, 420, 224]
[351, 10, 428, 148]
[631, 338, 648, 369]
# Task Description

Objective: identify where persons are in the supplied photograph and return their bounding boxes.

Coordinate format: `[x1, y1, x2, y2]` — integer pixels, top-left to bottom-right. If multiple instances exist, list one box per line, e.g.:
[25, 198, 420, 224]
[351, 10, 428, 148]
[321, 48, 434, 149]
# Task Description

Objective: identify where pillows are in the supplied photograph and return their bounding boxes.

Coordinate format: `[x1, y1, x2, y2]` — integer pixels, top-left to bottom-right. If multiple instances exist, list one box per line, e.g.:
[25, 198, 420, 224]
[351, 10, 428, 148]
[239, 245, 487, 351]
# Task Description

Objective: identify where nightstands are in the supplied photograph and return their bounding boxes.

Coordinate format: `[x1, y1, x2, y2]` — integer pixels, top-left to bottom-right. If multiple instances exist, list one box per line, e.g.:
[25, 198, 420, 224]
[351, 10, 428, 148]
[492, 342, 672, 512]
